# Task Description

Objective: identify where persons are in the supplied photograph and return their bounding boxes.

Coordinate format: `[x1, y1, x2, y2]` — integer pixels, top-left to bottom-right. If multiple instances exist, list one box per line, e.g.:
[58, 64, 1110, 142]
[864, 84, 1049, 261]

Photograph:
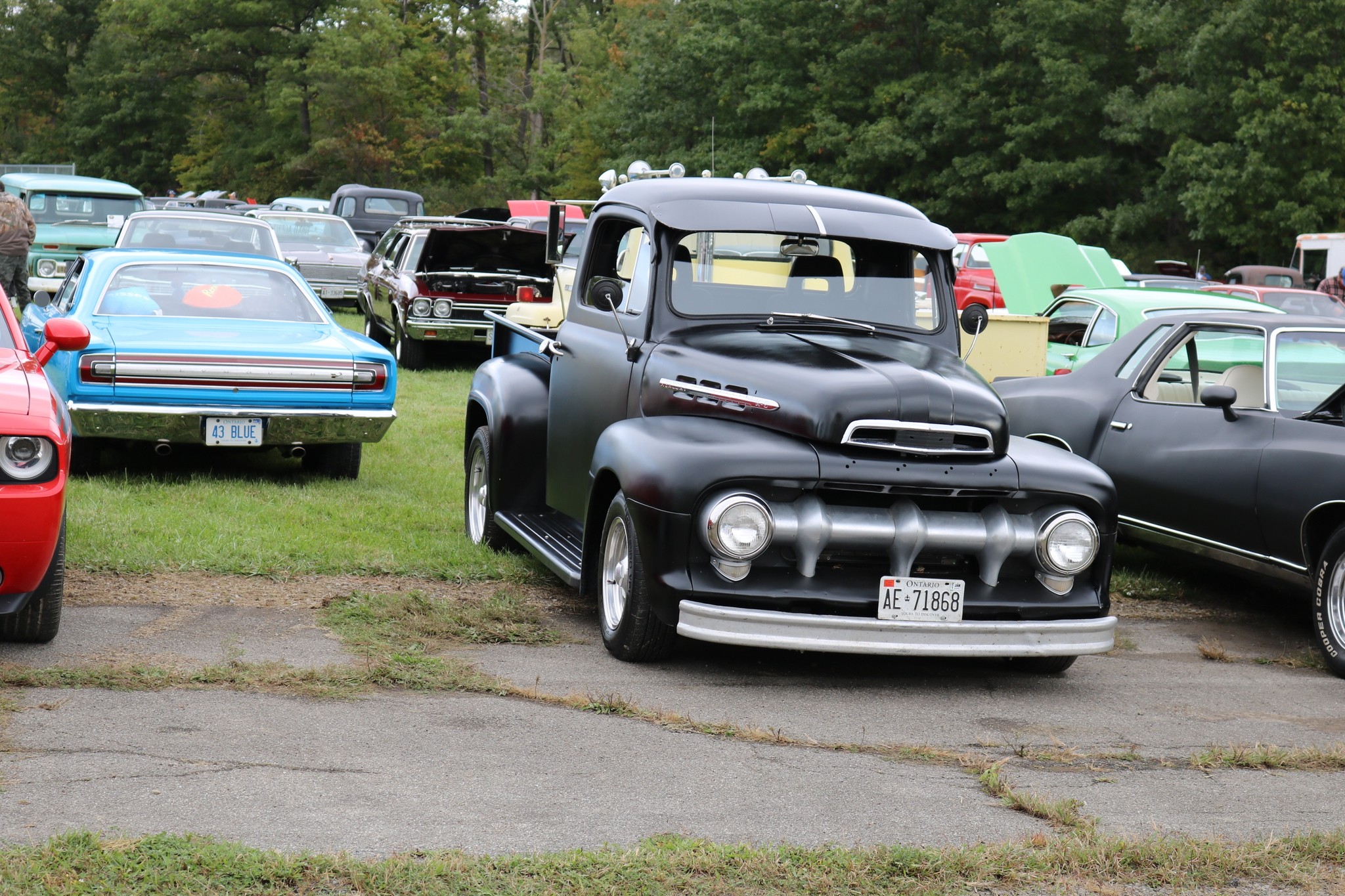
[227, 192, 238, 201]
[1195, 265, 1211, 280]
[1316, 267, 1345, 317]
[165, 190, 176, 197]
[0, 181, 37, 315]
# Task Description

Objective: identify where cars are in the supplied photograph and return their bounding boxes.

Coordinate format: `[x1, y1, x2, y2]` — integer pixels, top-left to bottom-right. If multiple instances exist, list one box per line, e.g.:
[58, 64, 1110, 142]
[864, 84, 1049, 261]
[0, 284, 91, 646]
[986, 314, 1345, 677]
[466, 178, 1118, 677]
[1, 172, 1345, 480]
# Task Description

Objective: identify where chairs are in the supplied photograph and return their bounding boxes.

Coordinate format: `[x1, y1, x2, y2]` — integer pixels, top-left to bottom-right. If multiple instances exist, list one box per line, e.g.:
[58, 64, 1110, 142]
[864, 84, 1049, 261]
[671, 244, 693, 308]
[224, 241, 255, 253]
[1216, 364, 1264, 408]
[142, 233, 176, 248]
[785, 255, 846, 317]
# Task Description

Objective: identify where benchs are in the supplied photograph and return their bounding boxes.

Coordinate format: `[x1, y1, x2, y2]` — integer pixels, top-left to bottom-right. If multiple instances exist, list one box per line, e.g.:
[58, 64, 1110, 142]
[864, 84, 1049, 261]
[1157, 381, 1212, 405]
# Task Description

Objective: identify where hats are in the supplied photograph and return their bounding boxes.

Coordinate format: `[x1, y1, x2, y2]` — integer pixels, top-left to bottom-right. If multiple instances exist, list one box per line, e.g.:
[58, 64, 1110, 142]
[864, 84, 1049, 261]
[228, 192, 238, 198]
